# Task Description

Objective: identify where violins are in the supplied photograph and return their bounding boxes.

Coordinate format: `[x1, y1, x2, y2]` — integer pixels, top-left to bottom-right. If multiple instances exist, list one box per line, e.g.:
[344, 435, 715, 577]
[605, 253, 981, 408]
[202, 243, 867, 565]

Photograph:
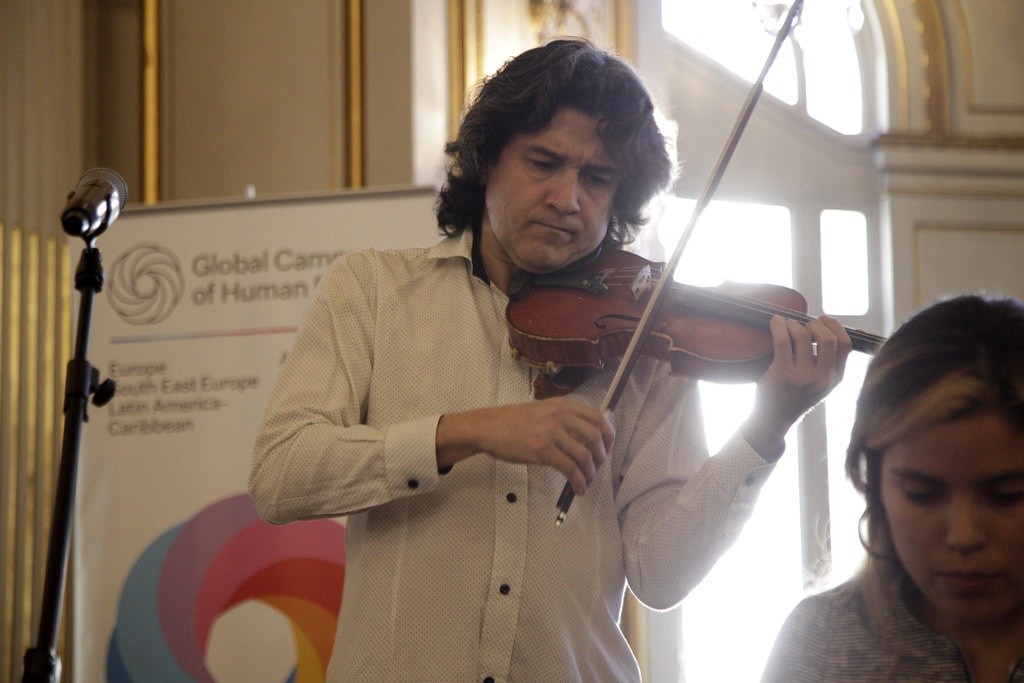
[504, 244, 891, 385]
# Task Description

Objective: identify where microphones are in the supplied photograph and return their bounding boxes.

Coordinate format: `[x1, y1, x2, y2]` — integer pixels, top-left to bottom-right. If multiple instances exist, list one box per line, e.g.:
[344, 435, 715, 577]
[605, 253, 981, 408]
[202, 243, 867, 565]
[60, 169, 128, 237]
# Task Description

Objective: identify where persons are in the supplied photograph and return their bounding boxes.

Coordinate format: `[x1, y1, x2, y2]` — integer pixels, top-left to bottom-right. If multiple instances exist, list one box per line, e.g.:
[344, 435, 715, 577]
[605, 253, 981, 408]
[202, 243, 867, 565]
[760, 295, 1023, 683]
[249, 35, 853, 683]
